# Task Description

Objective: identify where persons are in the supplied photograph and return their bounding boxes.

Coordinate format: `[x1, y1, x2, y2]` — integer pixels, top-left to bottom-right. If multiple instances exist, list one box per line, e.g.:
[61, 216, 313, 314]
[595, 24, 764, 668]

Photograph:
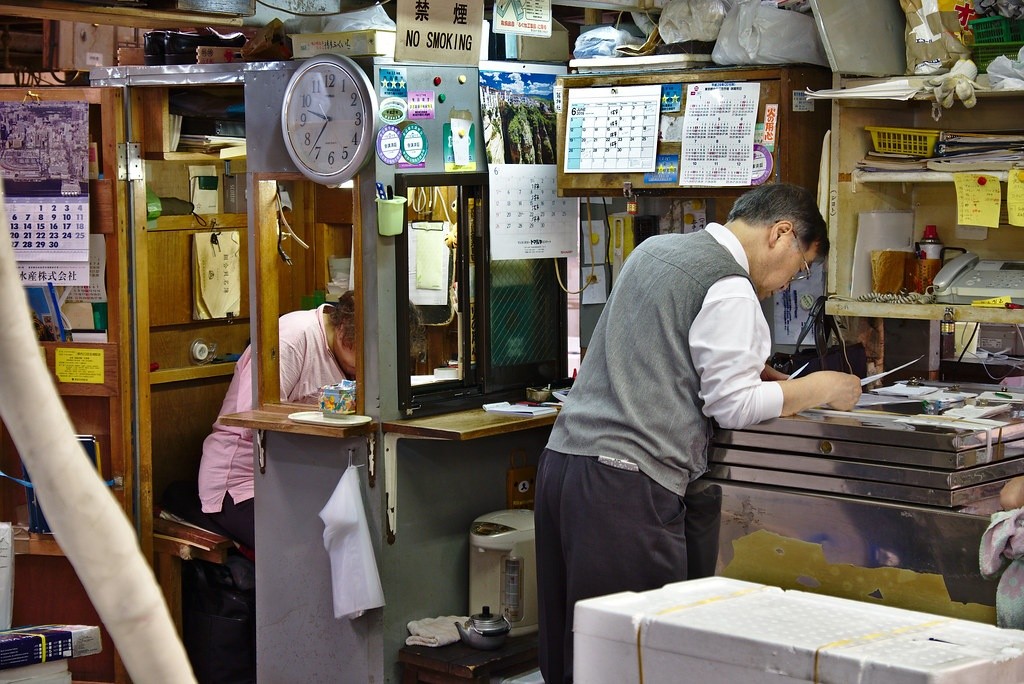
[535, 185, 861, 683]
[197, 287, 422, 684]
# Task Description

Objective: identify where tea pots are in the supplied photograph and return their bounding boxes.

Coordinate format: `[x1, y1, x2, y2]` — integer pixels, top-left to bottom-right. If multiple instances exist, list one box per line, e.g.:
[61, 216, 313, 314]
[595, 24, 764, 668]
[453, 606, 512, 649]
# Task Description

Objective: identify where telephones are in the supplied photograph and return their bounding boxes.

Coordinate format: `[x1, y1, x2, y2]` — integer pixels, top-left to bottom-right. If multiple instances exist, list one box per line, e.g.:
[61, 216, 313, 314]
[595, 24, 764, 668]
[931, 253, 1024, 304]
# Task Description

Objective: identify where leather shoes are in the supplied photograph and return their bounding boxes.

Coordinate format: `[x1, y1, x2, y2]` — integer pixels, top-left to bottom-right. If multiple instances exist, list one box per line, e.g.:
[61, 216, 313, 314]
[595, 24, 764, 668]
[143, 26, 246, 66]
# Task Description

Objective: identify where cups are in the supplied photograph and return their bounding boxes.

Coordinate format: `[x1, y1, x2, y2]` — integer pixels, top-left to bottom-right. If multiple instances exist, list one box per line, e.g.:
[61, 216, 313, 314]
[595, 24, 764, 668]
[955, 322, 980, 354]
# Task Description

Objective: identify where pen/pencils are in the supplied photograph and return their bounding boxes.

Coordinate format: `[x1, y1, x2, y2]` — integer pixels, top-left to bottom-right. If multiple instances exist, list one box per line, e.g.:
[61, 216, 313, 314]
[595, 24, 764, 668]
[992, 393, 1012, 399]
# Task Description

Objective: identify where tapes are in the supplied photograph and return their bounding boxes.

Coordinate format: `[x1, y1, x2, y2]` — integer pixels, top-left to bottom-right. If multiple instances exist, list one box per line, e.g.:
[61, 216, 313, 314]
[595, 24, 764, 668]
[193, 344, 208, 360]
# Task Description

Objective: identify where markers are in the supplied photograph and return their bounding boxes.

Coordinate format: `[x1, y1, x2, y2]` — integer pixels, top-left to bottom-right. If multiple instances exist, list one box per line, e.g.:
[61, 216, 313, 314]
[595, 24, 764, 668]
[920, 250, 926, 259]
[914, 241, 922, 258]
[387, 185, 393, 200]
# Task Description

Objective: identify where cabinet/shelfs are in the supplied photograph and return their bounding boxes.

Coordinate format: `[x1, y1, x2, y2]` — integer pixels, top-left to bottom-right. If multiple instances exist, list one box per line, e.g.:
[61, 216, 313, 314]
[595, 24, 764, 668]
[825, 73, 1024, 326]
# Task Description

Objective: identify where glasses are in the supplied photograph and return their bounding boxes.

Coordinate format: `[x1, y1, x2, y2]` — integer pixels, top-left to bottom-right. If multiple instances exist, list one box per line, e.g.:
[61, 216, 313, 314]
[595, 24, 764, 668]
[776, 219, 812, 282]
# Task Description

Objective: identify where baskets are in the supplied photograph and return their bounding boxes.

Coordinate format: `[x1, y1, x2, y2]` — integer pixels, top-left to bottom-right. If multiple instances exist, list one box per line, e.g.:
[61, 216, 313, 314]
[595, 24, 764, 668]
[865, 127, 941, 157]
[966, 15, 1024, 73]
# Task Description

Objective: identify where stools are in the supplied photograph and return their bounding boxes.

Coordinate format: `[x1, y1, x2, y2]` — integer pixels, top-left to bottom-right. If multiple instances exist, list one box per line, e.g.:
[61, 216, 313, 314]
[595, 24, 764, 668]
[398, 632, 540, 684]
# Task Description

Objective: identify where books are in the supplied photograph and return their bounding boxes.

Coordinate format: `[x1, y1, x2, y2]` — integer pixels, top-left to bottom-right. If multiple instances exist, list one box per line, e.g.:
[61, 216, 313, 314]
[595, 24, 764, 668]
[166, 109, 247, 162]
[924, 129, 1024, 176]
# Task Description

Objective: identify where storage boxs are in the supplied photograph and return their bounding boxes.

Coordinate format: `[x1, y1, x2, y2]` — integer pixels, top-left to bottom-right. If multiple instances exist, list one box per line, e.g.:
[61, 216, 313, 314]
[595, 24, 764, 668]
[504, 16, 569, 62]
[572, 574, 1024, 684]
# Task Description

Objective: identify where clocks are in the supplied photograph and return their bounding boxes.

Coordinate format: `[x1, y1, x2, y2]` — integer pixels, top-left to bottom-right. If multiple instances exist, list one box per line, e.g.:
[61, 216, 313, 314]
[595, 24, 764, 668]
[280, 52, 381, 187]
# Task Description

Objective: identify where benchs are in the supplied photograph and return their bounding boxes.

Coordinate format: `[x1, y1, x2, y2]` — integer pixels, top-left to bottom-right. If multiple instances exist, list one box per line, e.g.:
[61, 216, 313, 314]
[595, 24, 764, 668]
[151, 362, 240, 639]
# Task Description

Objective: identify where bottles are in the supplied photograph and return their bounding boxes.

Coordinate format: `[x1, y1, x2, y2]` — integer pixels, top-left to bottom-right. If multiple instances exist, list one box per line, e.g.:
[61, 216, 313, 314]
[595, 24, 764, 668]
[914, 224, 942, 259]
[941, 323, 955, 359]
